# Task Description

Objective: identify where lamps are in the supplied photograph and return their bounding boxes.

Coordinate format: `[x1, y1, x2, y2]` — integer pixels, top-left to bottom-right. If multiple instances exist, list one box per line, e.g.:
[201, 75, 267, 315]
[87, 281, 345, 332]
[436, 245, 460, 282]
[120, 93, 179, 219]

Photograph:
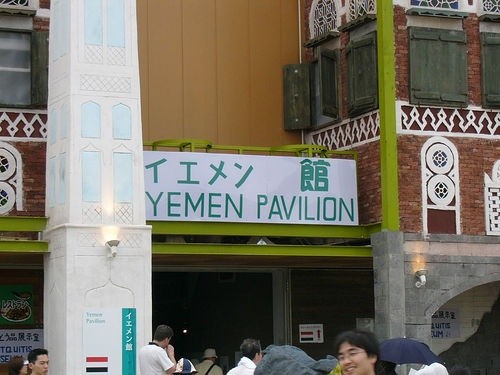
[105, 240, 119, 257]
[415, 269, 429, 288]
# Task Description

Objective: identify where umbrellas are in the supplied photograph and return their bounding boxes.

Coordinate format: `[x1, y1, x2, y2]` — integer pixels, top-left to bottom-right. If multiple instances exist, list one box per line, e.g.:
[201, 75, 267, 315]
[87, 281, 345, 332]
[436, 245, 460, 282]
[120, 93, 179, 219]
[378, 336, 442, 375]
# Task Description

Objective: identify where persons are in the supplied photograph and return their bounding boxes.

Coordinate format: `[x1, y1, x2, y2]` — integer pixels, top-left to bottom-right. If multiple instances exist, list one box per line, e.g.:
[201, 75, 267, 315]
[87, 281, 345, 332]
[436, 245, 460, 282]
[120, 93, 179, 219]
[136, 324, 176, 375]
[172, 329, 449, 375]
[225, 338, 262, 375]
[6, 355, 28, 375]
[27, 348, 49, 375]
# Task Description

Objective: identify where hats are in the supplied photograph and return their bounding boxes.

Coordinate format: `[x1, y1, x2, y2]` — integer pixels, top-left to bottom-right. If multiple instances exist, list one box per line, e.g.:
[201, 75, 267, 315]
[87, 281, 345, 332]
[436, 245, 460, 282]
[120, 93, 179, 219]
[172, 357, 198, 374]
[254, 345, 337, 375]
[203, 349, 217, 358]
